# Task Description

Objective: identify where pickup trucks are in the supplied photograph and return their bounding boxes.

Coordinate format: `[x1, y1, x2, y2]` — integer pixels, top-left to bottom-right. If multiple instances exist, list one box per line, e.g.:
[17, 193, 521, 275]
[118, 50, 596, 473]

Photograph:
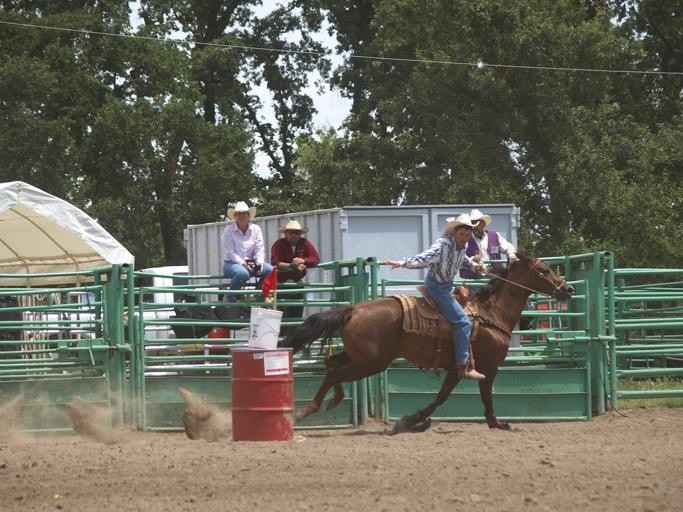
[23, 266, 188, 361]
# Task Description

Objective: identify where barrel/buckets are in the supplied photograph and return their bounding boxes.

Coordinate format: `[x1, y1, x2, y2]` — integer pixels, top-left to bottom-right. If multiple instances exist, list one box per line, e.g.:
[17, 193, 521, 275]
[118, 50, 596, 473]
[232, 347, 294, 441]
[249, 306, 284, 348]
[209, 328, 230, 364]
[176, 343, 205, 373]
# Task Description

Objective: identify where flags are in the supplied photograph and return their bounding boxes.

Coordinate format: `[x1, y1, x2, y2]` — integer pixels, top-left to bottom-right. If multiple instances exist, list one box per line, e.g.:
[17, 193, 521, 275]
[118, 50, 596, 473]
[261, 266, 277, 298]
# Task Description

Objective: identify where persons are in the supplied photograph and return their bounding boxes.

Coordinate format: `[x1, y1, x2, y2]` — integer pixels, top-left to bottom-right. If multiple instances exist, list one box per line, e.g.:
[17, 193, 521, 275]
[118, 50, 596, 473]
[382, 213, 490, 381]
[458, 209, 520, 278]
[221, 200, 274, 309]
[270, 220, 320, 339]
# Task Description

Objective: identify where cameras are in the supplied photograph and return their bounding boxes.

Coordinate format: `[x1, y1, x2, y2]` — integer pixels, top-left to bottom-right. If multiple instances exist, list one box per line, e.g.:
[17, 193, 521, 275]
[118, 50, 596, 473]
[246, 260, 261, 271]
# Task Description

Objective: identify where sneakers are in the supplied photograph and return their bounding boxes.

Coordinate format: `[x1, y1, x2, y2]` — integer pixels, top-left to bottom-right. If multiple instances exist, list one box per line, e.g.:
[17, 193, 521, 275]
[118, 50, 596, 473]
[456, 368, 486, 380]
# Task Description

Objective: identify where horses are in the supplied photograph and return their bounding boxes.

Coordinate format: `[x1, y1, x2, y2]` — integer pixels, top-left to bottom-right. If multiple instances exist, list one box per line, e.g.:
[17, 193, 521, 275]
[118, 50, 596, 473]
[277, 250, 576, 436]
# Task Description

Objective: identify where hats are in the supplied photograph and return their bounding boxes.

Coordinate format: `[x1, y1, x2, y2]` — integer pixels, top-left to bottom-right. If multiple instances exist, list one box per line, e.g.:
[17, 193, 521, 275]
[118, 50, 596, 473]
[443, 212, 481, 237]
[277, 219, 310, 234]
[227, 200, 256, 221]
[469, 208, 493, 226]
